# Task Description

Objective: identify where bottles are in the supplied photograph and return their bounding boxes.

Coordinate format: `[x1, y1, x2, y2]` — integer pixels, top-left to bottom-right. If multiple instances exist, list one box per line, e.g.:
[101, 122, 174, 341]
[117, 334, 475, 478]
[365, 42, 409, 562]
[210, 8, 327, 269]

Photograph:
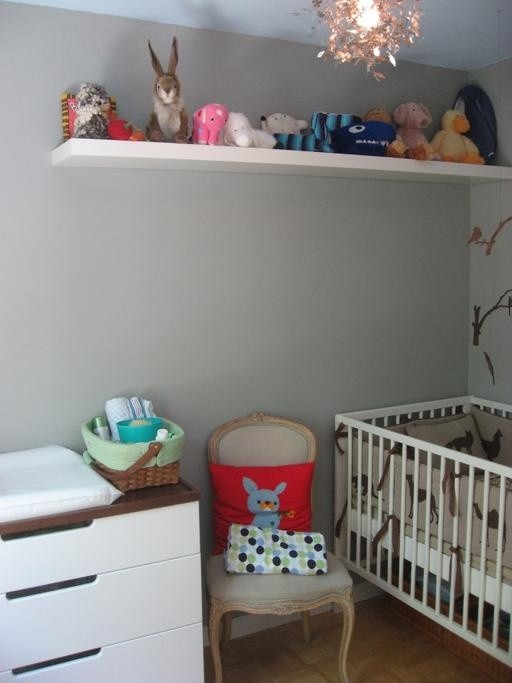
[155, 428, 168, 442]
[91, 416, 110, 440]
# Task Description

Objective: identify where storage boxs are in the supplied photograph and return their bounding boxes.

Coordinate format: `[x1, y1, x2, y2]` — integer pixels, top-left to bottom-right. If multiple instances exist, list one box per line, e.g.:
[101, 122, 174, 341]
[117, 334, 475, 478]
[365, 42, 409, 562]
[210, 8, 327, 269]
[443, 598, 512, 683]
[385, 569, 474, 647]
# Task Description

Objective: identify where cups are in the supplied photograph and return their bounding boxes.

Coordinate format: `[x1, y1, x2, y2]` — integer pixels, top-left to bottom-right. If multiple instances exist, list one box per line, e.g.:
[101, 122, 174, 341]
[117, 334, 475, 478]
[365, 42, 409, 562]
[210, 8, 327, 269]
[116, 416, 163, 443]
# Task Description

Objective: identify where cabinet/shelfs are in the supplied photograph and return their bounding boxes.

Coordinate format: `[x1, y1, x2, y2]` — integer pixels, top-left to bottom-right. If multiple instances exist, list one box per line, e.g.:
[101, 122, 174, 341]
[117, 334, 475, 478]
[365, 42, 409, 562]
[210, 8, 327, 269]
[0, 500, 204, 683]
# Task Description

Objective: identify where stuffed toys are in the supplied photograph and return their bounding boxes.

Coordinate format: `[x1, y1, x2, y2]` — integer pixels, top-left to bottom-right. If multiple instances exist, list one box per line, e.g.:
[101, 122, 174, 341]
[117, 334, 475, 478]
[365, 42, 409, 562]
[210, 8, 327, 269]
[73, 35, 485, 165]
[75, 36, 485, 166]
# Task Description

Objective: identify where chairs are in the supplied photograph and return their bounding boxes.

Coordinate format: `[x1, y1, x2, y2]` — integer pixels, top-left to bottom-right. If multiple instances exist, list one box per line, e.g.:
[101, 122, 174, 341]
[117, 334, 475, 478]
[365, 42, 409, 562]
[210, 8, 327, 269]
[208, 414, 356, 683]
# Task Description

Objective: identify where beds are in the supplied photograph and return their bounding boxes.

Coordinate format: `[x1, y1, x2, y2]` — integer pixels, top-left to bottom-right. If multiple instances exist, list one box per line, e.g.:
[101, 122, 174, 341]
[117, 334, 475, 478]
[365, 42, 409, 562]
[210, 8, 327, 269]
[335, 395, 512, 667]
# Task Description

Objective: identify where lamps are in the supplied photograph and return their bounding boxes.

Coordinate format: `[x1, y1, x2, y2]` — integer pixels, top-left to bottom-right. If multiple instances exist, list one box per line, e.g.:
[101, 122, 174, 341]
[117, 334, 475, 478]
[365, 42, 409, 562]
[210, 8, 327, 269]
[289, 0, 422, 83]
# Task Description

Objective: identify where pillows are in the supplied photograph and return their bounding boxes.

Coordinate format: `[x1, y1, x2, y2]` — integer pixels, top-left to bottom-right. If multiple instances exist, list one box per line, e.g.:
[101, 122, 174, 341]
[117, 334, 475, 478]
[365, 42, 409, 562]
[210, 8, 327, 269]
[208, 463, 315, 555]
[407, 415, 490, 474]
[0, 446, 124, 522]
[225, 523, 327, 575]
